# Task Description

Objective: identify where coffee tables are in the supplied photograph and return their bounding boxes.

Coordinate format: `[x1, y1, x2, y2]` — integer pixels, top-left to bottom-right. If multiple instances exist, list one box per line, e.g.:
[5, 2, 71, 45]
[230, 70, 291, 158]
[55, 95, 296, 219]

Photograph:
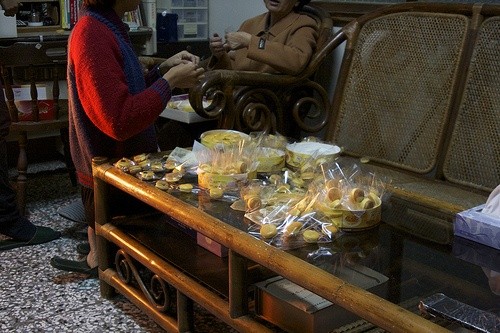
[92, 147, 500, 333]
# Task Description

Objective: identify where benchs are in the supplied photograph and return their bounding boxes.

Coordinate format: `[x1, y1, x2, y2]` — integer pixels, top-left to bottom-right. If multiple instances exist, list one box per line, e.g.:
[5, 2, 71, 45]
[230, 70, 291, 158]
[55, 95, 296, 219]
[188, 3, 500, 245]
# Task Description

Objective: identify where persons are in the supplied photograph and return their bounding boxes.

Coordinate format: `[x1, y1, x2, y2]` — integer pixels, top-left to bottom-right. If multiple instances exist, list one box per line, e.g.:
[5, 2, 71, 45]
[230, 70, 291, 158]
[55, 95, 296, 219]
[0, 0, 61, 250]
[59, 0, 320, 239]
[50, 0, 205, 279]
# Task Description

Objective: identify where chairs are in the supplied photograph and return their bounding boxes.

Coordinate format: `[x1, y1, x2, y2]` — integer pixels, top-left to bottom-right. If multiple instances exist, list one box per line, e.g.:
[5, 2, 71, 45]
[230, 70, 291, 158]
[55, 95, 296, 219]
[139, 4, 333, 129]
[0, 42, 77, 216]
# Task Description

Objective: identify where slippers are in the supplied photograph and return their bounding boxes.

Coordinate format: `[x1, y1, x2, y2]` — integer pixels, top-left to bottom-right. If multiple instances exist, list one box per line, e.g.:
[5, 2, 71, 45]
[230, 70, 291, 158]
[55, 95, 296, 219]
[77, 243, 90, 255]
[58, 198, 89, 223]
[0, 226, 61, 251]
[50, 256, 99, 275]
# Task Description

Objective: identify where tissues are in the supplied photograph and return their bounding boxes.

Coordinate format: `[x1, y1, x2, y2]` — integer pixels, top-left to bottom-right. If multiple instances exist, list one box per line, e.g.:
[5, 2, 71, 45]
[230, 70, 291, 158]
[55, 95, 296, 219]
[454, 184, 500, 249]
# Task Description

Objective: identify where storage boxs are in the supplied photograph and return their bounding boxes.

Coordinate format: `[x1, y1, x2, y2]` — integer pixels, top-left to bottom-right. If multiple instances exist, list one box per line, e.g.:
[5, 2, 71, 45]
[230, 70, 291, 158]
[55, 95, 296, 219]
[254, 260, 389, 333]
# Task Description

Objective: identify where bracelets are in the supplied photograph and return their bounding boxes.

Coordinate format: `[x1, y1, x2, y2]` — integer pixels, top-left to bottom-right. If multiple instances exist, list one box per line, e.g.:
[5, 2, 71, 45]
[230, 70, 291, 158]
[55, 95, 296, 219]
[157, 67, 161, 78]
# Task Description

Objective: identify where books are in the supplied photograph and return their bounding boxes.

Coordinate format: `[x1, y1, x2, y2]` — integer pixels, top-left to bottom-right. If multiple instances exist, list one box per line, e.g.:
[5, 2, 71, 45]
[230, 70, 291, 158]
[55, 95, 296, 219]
[60, 0, 81, 31]
[121, 0, 147, 30]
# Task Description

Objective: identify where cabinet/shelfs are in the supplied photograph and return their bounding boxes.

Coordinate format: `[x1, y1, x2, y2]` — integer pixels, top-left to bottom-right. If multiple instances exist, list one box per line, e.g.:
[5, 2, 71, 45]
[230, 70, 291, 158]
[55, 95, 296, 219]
[157, 0, 209, 44]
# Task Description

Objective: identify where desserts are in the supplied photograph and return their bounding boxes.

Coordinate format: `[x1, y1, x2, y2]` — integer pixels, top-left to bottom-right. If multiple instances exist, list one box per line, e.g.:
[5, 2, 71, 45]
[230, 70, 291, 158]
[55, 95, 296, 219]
[116, 131, 379, 244]
